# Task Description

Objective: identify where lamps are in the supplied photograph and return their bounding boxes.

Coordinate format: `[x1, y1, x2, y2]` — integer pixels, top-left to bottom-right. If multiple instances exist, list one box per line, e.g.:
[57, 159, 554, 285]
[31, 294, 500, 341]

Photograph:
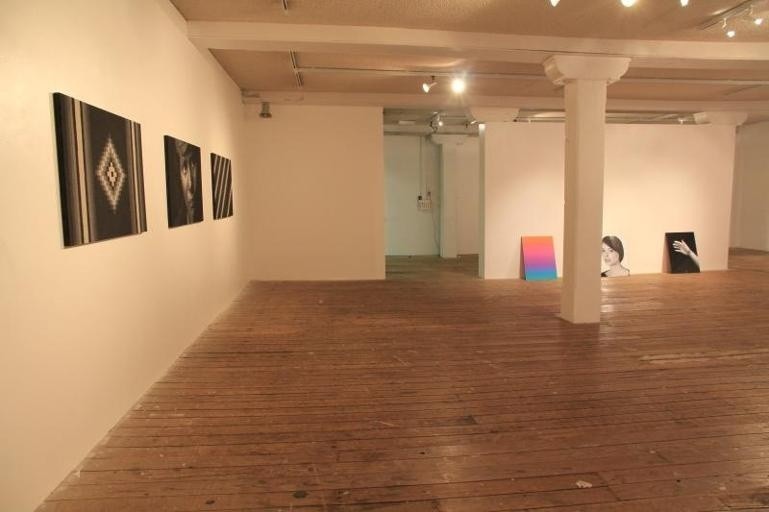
[422, 76, 437, 93]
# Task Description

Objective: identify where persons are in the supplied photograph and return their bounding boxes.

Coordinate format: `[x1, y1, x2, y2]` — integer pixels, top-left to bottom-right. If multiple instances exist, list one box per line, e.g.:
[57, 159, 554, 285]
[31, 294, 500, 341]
[168, 139, 202, 226]
[672, 240, 700, 270]
[600, 235, 631, 277]
